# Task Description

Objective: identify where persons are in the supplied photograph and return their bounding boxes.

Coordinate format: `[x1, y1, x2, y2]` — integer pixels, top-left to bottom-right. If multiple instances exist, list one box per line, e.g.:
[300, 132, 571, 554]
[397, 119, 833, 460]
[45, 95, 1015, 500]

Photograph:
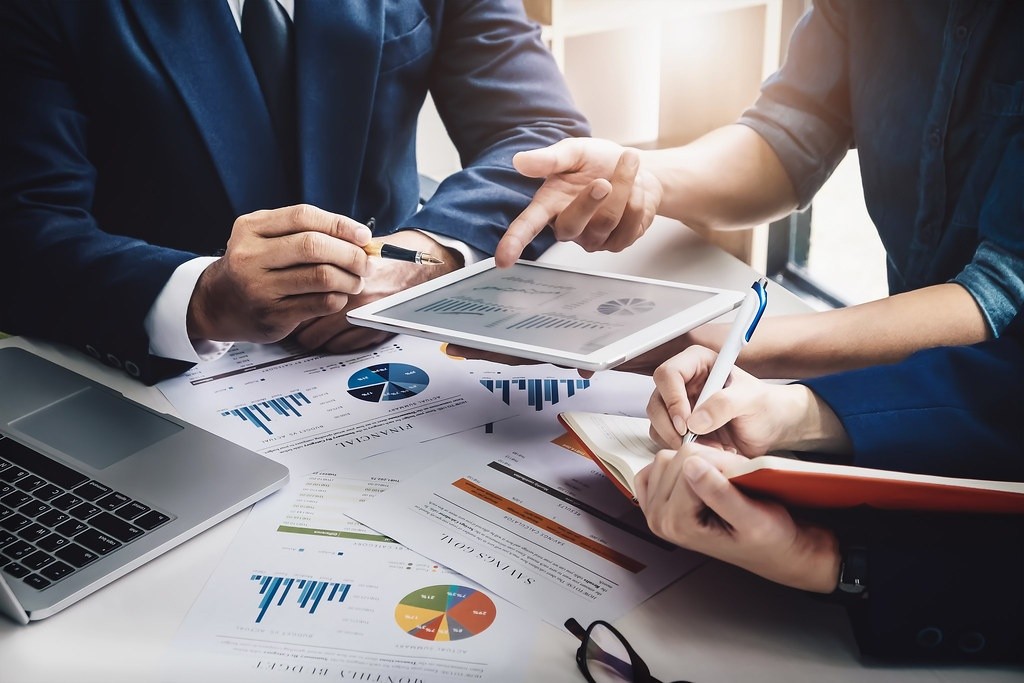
[496, 0, 1024, 379]
[0, 0, 593, 386]
[633, 303, 1023, 669]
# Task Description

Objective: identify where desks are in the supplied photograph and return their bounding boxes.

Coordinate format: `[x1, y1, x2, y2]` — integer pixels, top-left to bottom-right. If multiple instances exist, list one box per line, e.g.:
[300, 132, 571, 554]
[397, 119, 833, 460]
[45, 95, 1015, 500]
[1, 213, 1024, 682]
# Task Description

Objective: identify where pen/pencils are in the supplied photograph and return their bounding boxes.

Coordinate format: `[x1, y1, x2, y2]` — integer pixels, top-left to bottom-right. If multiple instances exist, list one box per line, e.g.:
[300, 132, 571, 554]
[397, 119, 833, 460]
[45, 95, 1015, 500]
[682, 277, 768, 452]
[337, 238, 445, 265]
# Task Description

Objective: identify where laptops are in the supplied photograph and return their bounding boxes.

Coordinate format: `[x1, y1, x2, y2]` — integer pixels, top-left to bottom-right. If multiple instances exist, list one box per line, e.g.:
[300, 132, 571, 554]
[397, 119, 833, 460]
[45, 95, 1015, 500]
[0, 346, 291, 625]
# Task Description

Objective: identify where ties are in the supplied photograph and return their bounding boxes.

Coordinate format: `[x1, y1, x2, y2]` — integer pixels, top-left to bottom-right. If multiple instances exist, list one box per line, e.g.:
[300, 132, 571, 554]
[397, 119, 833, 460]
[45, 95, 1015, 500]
[240, 0, 300, 204]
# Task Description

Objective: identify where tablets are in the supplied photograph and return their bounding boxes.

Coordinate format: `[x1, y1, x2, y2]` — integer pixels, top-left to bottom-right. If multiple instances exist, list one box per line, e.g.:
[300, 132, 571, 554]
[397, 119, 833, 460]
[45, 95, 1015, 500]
[346, 258, 750, 371]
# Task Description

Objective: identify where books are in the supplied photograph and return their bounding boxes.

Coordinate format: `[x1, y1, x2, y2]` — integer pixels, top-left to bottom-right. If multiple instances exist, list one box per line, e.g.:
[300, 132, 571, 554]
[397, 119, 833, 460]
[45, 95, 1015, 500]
[557, 412, 1024, 509]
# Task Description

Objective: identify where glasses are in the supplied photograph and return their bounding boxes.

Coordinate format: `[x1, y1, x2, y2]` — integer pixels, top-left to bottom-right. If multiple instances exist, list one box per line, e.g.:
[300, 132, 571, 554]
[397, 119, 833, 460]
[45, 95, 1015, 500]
[564, 618, 693, 683]
